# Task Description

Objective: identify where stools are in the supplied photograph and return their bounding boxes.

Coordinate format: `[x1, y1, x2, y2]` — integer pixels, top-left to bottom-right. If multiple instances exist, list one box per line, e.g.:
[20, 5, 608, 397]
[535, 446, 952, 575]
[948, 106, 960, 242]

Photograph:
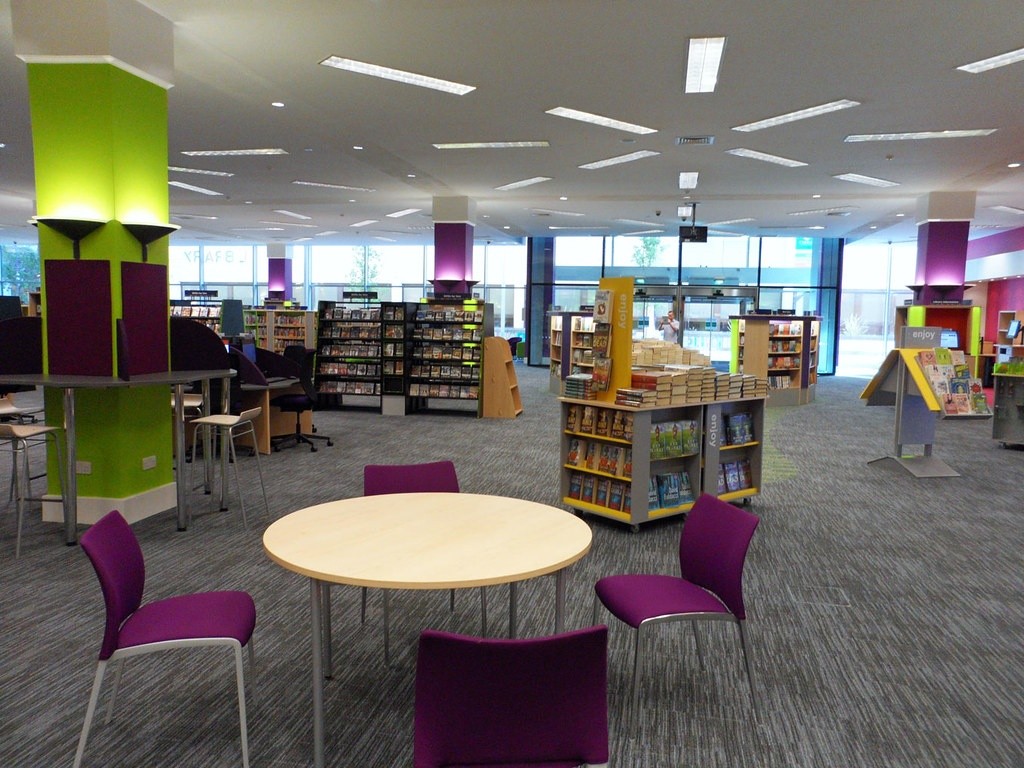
[0, 397, 67, 559]
[172, 393, 268, 529]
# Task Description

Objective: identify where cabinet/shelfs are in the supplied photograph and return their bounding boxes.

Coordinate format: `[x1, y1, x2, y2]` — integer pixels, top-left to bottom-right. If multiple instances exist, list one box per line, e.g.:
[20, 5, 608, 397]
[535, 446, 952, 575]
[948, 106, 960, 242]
[546, 316, 822, 533]
[170, 303, 522, 420]
[994, 310, 1024, 347]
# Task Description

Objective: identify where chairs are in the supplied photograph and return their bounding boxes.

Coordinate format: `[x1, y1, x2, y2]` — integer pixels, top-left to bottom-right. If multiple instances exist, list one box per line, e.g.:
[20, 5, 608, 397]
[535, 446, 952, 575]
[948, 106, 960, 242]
[413, 624, 609, 768]
[360, 461, 487, 669]
[72, 512, 257, 768]
[591, 492, 762, 724]
[507, 337, 525, 360]
[270, 346, 334, 453]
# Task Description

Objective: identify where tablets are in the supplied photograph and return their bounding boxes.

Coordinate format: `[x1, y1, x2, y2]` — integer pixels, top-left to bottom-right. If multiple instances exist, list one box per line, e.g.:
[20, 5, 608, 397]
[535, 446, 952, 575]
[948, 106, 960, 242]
[662, 316, 668, 325]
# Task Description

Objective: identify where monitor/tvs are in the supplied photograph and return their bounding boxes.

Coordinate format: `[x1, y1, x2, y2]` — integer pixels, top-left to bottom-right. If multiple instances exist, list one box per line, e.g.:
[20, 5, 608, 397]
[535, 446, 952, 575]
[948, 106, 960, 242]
[1006, 320, 1021, 339]
[679, 226, 707, 242]
[940, 330, 960, 348]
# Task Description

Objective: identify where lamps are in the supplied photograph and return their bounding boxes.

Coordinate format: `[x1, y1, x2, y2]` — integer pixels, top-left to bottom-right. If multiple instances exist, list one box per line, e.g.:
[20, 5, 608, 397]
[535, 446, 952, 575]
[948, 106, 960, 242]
[28, 216, 181, 263]
[906, 285, 973, 301]
[428, 280, 479, 293]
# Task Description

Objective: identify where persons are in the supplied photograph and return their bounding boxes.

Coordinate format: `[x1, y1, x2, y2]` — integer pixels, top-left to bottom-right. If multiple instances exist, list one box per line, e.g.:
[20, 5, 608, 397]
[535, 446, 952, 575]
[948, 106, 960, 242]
[658, 310, 681, 345]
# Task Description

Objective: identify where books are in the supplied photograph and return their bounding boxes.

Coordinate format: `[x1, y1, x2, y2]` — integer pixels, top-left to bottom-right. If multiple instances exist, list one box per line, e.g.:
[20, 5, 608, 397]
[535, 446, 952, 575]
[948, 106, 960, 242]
[171, 302, 485, 400]
[919, 344, 988, 416]
[738, 318, 817, 389]
[561, 365, 770, 514]
[630, 337, 711, 368]
[546, 290, 616, 392]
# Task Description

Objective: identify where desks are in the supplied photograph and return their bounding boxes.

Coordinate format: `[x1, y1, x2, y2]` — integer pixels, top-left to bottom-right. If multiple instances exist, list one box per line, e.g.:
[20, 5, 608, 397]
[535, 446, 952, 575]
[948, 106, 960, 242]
[979, 354, 996, 388]
[0, 368, 238, 547]
[263, 492, 592, 768]
[240, 376, 315, 455]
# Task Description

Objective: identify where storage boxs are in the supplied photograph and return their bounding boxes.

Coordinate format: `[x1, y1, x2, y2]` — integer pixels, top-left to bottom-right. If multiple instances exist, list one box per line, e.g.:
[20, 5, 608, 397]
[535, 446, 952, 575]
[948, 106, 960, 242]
[983, 342, 994, 354]
